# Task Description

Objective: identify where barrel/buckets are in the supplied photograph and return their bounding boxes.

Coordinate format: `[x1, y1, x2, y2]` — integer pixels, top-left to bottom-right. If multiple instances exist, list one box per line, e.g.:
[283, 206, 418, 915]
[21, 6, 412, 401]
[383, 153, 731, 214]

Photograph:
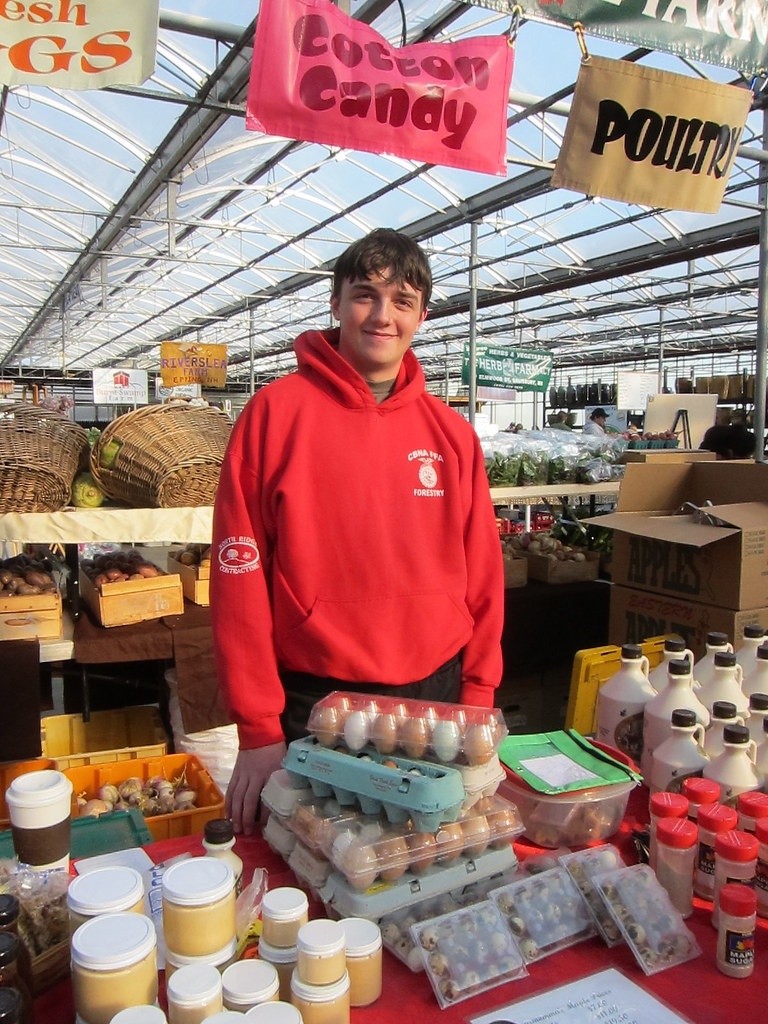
[676, 374, 757, 400]
[550, 383, 617, 407]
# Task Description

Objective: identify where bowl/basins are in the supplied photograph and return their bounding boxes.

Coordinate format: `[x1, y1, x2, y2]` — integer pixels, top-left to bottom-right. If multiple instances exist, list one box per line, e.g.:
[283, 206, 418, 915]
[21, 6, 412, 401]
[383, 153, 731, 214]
[498, 738, 638, 848]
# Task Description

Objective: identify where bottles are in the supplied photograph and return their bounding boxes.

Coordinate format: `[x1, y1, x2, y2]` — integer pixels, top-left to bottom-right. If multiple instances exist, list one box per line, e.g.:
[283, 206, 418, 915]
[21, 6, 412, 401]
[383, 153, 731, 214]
[597, 626, 768, 808]
[0, 893, 33, 1024]
[64, 817, 383, 1024]
[647, 778, 768, 977]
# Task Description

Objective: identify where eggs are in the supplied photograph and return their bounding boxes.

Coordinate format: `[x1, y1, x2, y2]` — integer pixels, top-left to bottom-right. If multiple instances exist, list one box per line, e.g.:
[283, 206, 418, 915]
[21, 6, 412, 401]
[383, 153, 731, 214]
[293, 699, 514, 890]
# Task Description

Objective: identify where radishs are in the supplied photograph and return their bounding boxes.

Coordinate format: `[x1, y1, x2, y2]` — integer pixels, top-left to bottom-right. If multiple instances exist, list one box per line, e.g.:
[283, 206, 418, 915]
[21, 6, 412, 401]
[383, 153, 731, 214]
[75, 775, 201, 822]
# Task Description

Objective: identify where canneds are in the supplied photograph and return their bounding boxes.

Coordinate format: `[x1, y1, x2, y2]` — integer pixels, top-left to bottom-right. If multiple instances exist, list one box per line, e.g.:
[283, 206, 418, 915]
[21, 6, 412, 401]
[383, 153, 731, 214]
[64, 856, 382, 1024]
[648, 777, 768, 978]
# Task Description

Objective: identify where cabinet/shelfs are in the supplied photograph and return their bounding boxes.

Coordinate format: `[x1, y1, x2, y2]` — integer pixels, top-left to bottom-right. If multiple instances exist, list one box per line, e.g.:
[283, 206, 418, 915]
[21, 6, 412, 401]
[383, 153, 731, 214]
[542, 371, 754, 432]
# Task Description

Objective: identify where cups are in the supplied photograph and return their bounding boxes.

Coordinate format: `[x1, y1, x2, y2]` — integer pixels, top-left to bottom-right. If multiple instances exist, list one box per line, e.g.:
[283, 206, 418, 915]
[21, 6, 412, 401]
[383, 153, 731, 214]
[5, 771, 73, 878]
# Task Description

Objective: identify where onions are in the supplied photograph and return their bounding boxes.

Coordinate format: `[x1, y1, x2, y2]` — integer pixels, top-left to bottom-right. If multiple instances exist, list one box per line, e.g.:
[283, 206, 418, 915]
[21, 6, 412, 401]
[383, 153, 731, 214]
[501, 532, 586, 566]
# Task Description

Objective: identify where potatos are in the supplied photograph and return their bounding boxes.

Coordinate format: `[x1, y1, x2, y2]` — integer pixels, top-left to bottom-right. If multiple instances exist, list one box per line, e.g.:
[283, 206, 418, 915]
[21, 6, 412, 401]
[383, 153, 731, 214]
[0, 544, 211, 600]
[622, 430, 676, 441]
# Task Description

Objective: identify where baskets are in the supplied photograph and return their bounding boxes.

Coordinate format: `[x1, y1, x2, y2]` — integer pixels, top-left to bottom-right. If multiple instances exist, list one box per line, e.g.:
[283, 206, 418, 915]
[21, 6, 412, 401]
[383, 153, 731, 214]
[86, 396, 235, 509]
[0, 377, 91, 513]
[626, 437, 680, 449]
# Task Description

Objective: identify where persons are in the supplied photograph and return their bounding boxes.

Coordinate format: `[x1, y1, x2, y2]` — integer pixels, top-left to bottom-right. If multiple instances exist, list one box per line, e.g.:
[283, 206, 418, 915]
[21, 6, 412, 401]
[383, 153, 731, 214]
[552, 411, 571, 431]
[581, 408, 610, 434]
[209, 229, 504, 836]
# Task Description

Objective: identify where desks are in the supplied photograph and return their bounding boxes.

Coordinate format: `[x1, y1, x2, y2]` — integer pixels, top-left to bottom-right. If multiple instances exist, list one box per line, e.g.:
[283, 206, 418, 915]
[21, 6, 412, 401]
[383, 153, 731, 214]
[0, 785, 768, 1024]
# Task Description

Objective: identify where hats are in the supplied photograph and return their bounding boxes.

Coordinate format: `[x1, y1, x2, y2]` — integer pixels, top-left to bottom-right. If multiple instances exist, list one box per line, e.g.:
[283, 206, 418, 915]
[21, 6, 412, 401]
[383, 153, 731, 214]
[591, 408, 609, 418]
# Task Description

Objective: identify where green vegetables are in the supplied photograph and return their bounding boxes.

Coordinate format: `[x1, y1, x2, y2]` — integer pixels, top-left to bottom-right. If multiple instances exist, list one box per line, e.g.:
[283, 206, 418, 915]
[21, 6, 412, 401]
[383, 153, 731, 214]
[553, 508, 617, 553]
[482, 441, 626, 489]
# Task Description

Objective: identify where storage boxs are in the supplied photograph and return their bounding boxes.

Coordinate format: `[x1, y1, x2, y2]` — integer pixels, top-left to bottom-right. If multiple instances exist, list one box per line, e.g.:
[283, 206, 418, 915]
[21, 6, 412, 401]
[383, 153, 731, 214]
[166, 550, 210, 607]
[60, 753, 226, 844]
[496, 439, 768, 738]
[0, 804, 154, 866]
[0, 578, 63, 641]
[38, 704, 169, 768]
[0, 758, 59, 829]
[77, 557, 184, 630]
